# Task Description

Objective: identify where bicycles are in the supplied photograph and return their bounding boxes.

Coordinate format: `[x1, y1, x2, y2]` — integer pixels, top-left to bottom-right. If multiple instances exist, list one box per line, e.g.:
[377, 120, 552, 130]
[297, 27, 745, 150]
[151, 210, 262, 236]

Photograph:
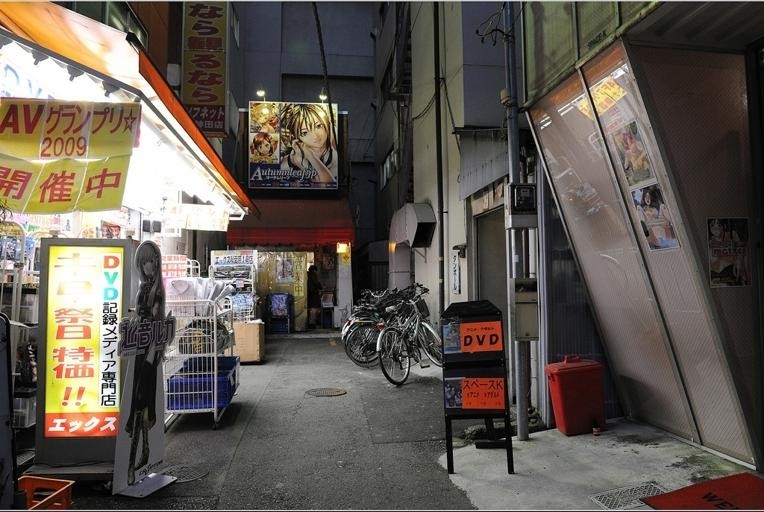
[339, 283, 442, 387]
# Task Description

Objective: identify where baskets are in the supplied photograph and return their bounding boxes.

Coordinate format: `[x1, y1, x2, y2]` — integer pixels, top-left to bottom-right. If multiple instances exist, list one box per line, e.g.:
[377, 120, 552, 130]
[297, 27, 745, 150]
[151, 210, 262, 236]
[167, 356, 239, 409]
[272, 319, 288, 332]
[404, 298, 429, 324]
[16, 475, 75, 510]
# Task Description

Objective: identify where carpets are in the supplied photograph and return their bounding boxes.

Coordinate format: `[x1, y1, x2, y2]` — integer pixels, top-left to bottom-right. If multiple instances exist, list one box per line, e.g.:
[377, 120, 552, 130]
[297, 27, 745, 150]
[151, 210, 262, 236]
[640, 472, 764, 510]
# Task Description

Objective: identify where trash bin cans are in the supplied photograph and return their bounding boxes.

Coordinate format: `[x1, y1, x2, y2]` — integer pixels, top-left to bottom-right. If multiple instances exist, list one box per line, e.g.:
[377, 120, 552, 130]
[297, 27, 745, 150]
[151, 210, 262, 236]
[546, 354, 606, 436]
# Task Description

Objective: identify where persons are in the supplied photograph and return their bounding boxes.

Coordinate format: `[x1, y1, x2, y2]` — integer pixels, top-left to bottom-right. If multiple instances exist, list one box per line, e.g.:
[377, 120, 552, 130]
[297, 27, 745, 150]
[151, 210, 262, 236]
[307, 264, 324, 330]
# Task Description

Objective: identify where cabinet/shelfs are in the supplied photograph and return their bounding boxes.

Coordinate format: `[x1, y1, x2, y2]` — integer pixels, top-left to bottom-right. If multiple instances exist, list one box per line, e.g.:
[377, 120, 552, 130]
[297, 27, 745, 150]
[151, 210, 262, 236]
[164, 297, 241, 429]
